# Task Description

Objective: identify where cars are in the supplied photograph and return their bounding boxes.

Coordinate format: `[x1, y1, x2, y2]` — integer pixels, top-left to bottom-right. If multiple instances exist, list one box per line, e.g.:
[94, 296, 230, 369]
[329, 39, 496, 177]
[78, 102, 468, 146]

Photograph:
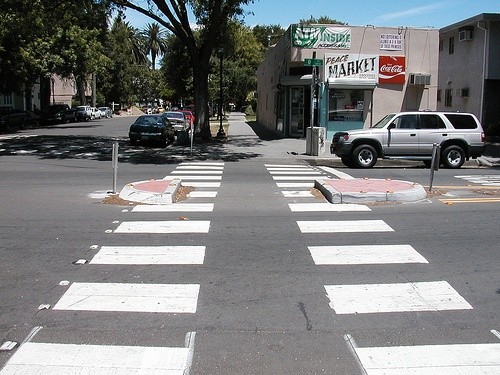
[128, 114, 176, 147]
[140, 104, 214, 132]
[0, 109, 34, 133]
[76, 105, 113, 122]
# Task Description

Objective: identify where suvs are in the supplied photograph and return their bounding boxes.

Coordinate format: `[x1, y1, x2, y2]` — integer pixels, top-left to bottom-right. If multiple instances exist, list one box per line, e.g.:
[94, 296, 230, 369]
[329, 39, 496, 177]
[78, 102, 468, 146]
[37, 104, 79, 127]
[329, 109, 486, 169]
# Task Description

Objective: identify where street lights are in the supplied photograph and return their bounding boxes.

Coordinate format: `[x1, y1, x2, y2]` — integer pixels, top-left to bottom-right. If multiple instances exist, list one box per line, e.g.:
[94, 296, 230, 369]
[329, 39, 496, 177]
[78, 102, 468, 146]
[216, 46, 226, 143]
